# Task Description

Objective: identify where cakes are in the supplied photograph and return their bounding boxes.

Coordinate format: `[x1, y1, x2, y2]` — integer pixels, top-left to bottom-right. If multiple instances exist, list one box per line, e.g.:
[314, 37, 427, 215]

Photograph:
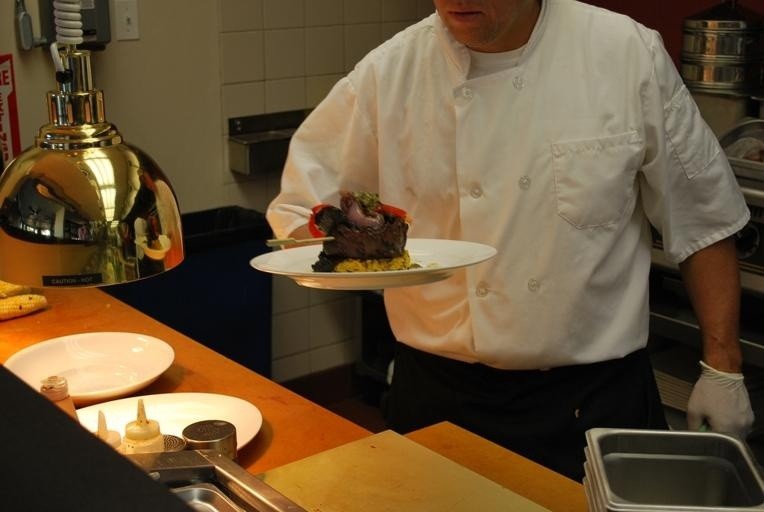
[308, 191, 412, 272]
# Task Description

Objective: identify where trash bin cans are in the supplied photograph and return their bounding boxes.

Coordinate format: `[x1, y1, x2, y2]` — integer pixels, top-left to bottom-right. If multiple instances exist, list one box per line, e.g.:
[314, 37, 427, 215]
[106, 205, 271, 381]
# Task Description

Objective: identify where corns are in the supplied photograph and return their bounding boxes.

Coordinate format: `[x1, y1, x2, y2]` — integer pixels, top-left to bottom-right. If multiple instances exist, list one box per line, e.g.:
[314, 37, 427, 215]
[0, 294, 48, 321]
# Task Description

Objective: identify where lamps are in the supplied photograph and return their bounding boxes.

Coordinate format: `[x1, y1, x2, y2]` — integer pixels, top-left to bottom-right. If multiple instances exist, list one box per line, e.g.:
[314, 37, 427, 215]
[1, 0, 187, 292]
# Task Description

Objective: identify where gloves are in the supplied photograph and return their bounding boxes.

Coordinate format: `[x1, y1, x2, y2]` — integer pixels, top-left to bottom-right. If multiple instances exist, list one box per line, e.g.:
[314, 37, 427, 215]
[688, 359, 755, 440]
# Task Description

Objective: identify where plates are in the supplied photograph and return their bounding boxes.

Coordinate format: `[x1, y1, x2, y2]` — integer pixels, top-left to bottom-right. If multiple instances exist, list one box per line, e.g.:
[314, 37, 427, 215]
[253, 235, 499, 292]
[579, 424, 762, 510]
[2, 334, 262, 475]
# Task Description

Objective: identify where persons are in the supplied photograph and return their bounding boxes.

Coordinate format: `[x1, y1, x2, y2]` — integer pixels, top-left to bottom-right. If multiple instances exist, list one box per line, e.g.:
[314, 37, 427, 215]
[265, 0, 754, 483]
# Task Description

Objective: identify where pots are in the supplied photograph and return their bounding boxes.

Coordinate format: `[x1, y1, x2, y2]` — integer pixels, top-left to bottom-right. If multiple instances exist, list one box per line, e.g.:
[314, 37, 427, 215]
[674, 3, 750, 92]
